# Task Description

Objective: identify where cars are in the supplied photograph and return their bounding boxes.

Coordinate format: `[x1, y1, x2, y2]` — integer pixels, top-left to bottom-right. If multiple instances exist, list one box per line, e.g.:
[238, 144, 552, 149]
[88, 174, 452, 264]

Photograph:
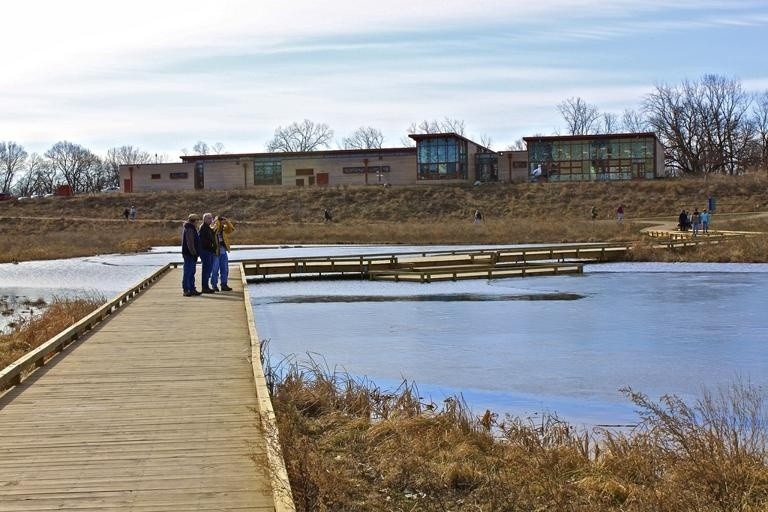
[16, 192, 54, 200]
[101, 184, 121, 193]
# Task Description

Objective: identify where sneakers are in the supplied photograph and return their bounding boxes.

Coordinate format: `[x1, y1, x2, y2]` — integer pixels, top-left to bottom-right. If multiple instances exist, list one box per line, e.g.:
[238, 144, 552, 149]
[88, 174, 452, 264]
[202, 287, 219, 293]
[221, 285, 232, 291]
[183, 287, 201, 297]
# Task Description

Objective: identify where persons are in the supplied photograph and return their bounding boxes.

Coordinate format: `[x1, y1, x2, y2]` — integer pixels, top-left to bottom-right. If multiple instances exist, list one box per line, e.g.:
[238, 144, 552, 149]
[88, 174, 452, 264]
[591, 207, 598, 223]
[678, 208, 710, 234]
[122, 206, 136, 220]
[616, 203, 625, 223]
[473, 209, 481, 224]
[324, 209, 330, 225]
[182, 212, 236, 297]
